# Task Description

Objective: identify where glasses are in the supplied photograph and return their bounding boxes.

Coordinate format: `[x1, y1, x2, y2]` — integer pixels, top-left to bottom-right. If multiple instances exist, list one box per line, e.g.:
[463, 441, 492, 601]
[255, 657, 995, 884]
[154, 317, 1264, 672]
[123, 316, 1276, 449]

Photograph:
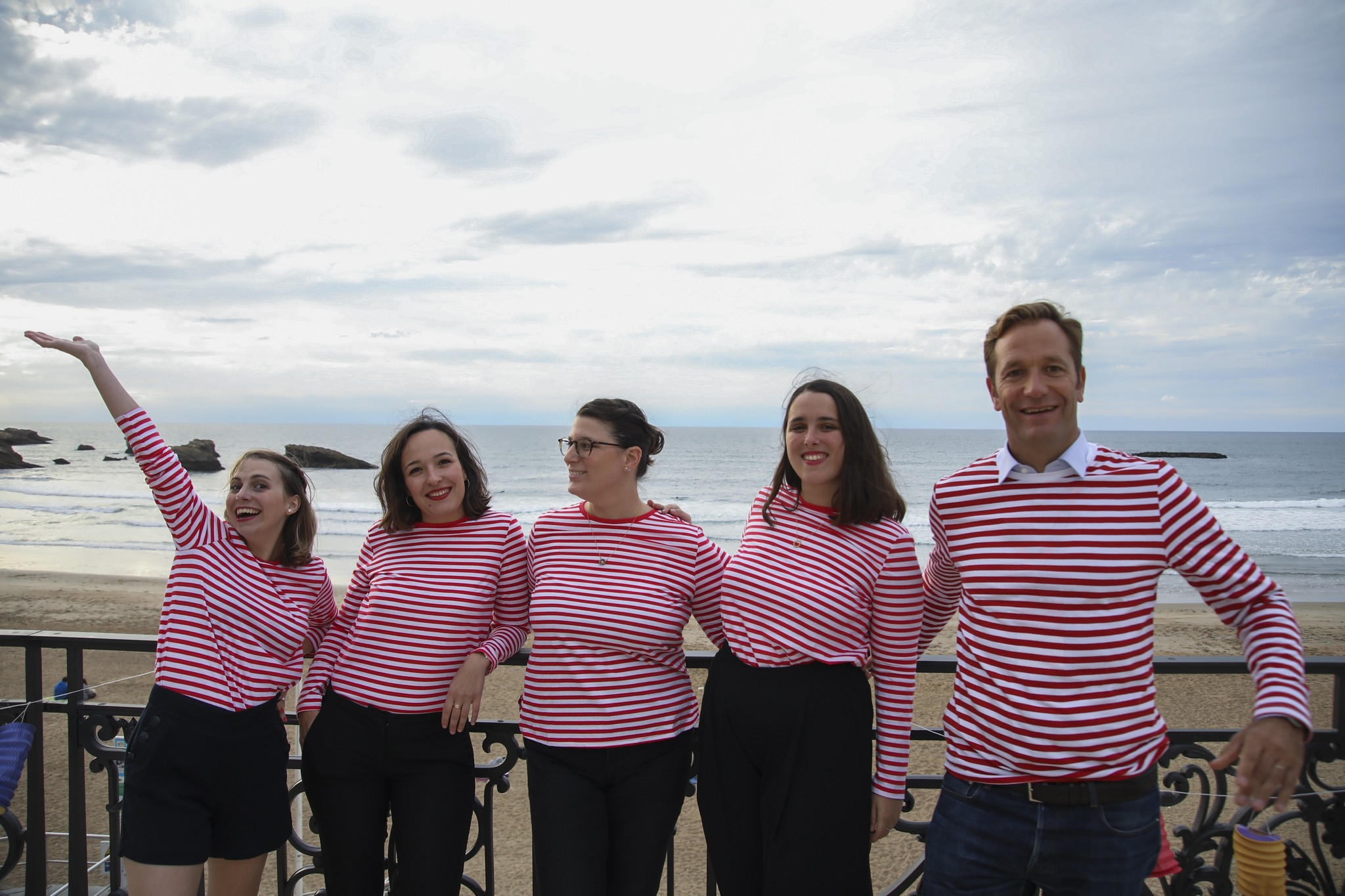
[558, 436, 628, 460]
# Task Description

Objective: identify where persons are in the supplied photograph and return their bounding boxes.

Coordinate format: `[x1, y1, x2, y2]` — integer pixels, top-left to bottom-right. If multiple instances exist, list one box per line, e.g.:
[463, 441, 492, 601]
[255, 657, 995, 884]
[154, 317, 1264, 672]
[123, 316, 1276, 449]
[645, 380, 922, 896]
[83, 678, 97, 700]
[53, 676, 69, 700]
[861, 303, 1315, 896]
[24, 331, 343, 894]
[298, 407, 527, 896]
[524, 398, 734, 896]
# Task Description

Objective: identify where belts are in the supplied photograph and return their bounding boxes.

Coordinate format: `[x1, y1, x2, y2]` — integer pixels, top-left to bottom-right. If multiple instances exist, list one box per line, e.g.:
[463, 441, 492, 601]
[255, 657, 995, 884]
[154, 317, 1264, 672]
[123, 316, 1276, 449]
[965, 762, 1158, 805]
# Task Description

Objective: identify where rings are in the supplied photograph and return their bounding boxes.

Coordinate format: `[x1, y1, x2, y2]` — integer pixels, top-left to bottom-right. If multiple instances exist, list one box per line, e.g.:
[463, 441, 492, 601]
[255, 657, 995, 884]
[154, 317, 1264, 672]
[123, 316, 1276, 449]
[1272, 764, 1289, 774]
[452, 703, 462, 710]
[281, 696, 286, 701]
